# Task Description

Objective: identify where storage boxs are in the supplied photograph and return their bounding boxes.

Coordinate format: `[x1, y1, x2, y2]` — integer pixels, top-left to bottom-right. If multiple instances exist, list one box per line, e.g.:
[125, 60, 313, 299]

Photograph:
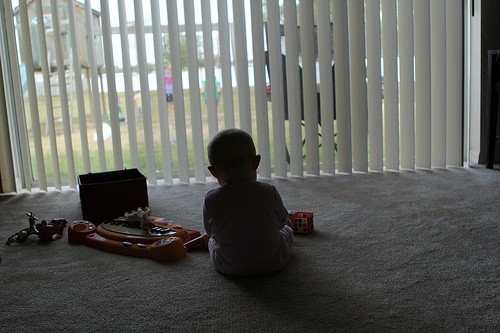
[77, 167, 150, 224]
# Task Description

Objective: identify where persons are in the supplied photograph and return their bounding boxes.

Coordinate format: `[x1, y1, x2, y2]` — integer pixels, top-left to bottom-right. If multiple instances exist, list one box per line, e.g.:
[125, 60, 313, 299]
[204, 128, 293, 276]
